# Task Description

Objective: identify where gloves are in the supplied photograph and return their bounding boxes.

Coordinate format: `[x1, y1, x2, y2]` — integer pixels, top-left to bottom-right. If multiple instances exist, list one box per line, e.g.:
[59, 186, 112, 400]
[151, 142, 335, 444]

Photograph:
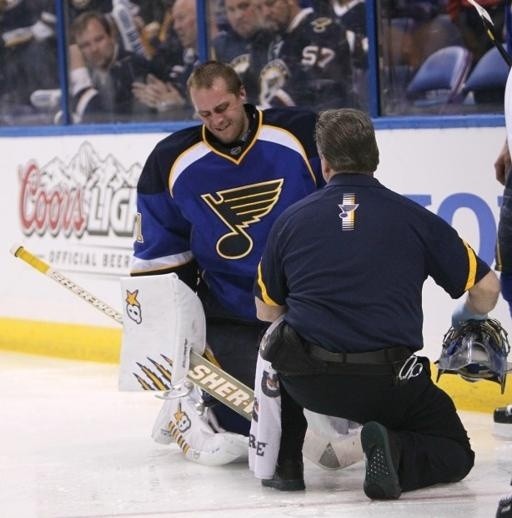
[451, 302, 489, 324]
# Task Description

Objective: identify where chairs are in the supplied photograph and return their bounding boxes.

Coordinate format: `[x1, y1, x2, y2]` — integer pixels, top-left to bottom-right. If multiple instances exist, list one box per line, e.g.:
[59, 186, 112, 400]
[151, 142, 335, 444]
[385, 43, 511, 116]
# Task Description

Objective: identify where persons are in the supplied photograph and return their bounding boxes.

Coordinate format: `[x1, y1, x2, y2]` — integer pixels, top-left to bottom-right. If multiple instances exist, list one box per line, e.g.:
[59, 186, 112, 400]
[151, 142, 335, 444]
[120, 60, 353, 468]
[471, 1, 511, 518]
[247, 107, 499, 500]
[1, 1, 512, 122]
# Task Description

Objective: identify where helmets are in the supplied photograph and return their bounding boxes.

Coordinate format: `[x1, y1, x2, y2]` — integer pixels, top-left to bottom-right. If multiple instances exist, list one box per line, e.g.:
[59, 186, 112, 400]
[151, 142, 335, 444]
[434, 317, 512, 395]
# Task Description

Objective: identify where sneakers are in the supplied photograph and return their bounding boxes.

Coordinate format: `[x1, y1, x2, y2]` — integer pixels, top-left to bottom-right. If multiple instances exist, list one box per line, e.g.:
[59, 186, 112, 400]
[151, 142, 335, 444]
[360, 421, 406, 501]
[262, 456, 308, 491]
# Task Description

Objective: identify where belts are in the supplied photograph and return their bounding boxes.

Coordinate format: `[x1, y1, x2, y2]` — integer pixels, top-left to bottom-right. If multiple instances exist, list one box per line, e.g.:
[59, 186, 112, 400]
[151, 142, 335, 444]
[307, 343, 409, 367]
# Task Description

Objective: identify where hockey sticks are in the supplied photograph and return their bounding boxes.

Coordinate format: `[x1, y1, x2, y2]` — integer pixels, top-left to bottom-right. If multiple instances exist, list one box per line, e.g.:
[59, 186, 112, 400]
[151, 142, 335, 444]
[467, 0, 510, 65]
[9, 243, 365, 470]
